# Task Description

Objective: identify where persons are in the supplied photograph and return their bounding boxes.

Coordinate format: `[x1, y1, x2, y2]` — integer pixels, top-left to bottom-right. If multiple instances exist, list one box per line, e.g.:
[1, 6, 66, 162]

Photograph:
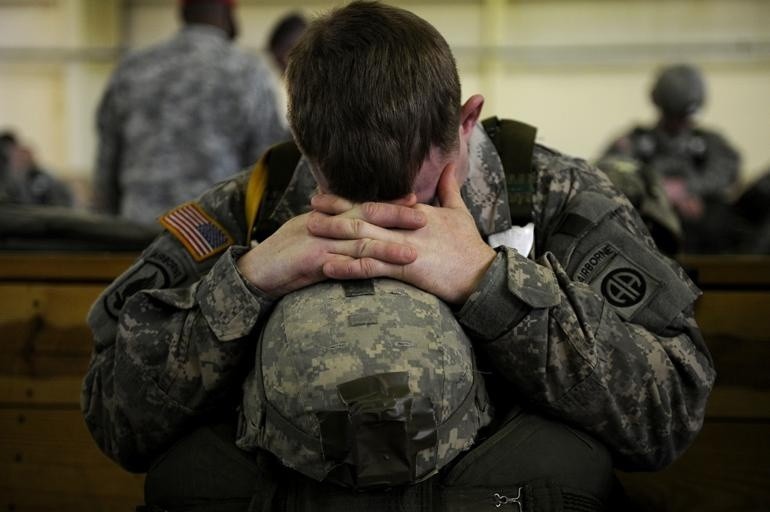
[87, 0, 289, 219]
[601, 62, 740, 196]
[266, 10, 310, 75]
[79, 0, 720, 511]
[662, 171, 770, 255]
[0, 132, 76, 207]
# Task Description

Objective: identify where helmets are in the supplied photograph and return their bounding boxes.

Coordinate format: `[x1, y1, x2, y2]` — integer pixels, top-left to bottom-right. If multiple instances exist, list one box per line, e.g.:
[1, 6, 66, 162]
[235, 278, 495, 490]
[652, 66, 704, 117]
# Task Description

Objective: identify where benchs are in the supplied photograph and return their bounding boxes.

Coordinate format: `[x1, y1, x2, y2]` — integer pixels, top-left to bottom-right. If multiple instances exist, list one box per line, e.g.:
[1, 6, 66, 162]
[1, 251, 770, 509]
